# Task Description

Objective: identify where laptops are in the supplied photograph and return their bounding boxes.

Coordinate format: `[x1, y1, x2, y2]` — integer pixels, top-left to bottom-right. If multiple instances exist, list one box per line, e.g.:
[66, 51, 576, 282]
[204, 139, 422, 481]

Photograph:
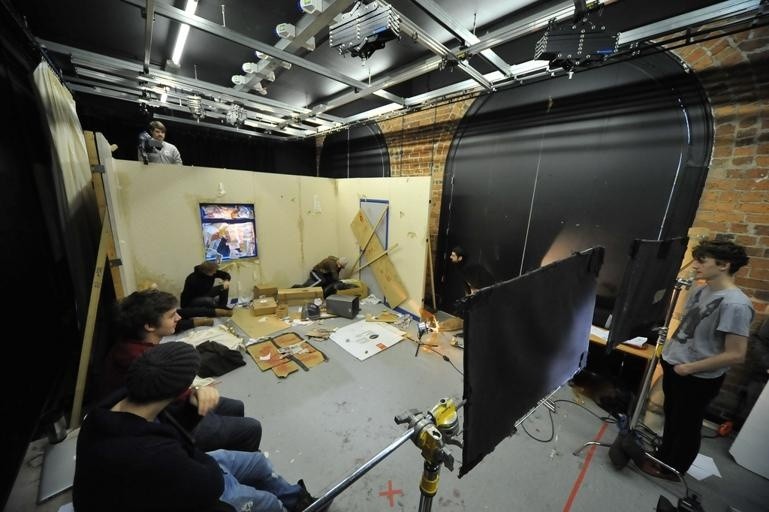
[36, 439, 77, 505]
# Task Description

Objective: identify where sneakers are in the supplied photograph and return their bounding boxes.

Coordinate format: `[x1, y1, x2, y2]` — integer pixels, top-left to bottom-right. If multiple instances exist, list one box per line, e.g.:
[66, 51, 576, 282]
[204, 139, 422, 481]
[640, 462, 684, 482]
[215, 309, 233, 316]
[282, 480, 335, 511]
[193, 317, 214, 328]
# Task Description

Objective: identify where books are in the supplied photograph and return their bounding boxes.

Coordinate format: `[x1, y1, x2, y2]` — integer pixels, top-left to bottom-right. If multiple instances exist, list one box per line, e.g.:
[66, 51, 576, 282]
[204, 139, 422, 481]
[623, 335, 648, 349]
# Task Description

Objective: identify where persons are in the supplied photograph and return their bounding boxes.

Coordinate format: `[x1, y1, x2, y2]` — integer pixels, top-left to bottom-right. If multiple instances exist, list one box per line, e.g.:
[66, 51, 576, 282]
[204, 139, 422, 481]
[304, 255, 348, 287]
[96, 288, 262, 451]
[72, 342, 332, 512]
[173, 307, 234, 333]
[645, 237, 755, 480]
[138, 122, 182, 167]
[180, 263, 233, 310]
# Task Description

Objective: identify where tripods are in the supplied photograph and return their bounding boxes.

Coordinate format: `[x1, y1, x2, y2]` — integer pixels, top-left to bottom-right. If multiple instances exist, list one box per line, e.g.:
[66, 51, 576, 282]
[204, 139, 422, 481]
[402, 332, 439, 357]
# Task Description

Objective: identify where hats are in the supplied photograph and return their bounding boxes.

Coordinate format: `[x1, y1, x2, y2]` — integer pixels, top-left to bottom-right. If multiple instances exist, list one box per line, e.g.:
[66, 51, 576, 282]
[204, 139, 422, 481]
[337, 257, 348, 268]
[125, 341, 200, 399]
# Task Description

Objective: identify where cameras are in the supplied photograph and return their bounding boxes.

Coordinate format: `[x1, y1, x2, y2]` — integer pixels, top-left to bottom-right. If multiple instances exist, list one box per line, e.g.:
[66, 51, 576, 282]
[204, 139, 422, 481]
[136, 130, 163, 165]
